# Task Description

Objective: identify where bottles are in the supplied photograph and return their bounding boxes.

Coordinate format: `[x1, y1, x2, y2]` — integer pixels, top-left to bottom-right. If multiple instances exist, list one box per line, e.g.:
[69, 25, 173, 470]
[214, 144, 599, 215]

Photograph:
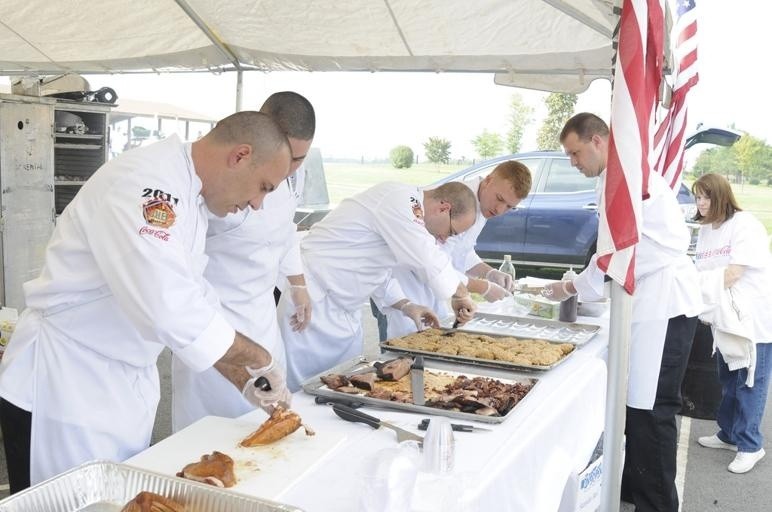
[557, 265, 579, 323]
[498, 254, 516, 295]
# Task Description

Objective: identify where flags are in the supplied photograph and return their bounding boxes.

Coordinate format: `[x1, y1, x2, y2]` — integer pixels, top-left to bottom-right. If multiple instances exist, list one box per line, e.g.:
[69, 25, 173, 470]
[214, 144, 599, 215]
[594, 0, 665, 298]
[650, 0, 700, 192]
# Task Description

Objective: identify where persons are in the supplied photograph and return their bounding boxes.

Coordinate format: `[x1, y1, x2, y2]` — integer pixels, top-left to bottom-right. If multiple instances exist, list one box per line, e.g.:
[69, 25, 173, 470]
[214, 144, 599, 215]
[689, 170, 772, 473]
[371, 159, 533, 354]
[168, 90, 316, 429]
[280, 179, 476, 390]
[0, 111, 291, 497]
[536, 112, 706, 511]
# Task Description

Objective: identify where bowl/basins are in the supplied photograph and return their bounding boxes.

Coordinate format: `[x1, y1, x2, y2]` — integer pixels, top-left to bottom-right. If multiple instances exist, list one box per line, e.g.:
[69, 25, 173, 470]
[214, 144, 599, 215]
[575, 297, 609, 317]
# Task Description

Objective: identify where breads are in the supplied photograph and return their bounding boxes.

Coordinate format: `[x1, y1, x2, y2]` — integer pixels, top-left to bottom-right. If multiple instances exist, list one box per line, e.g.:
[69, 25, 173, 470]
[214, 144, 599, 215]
[389, 327, 573, 367]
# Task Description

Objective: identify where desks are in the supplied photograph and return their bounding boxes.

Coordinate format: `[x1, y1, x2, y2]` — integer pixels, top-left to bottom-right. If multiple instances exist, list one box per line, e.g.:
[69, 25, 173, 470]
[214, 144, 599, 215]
[123, 278, 610, 510]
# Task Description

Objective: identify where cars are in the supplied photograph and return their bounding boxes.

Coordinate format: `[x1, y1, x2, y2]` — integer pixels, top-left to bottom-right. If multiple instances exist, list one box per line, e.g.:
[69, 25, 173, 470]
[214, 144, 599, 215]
[423, 128, 739, 282]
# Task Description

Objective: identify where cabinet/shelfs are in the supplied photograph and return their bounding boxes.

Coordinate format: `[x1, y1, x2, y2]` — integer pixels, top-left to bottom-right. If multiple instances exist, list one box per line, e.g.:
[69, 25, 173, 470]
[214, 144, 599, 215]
[0, 92, 118, 315]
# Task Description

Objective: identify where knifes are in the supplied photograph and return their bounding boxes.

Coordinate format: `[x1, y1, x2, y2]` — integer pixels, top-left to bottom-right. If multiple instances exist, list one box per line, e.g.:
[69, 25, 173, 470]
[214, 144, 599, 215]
[409, 356, 426, 407]
[330, 402, 425, 448]
[315, 395, 435, 415]
[253, 374, 294, 409]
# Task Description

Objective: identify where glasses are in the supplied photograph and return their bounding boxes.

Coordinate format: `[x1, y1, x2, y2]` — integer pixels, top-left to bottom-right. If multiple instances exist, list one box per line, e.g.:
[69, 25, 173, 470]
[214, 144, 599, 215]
[448, 209, 457, 237]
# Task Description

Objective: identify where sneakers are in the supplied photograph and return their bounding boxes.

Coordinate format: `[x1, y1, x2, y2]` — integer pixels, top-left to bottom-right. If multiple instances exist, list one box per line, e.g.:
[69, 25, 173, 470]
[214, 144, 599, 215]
[697, 433, 738, 451]
[727, 448, 765, 474]
